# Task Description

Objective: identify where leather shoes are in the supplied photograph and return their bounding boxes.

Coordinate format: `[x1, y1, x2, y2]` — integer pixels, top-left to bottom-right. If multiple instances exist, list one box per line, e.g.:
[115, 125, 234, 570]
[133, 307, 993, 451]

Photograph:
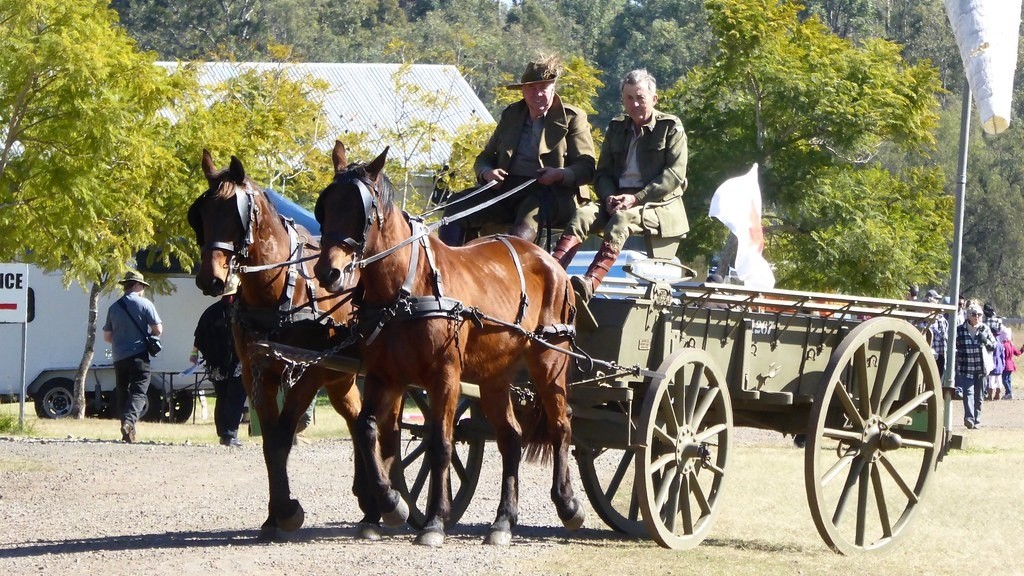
[219, 436, 243, 447]
[121, 421, 137, 445]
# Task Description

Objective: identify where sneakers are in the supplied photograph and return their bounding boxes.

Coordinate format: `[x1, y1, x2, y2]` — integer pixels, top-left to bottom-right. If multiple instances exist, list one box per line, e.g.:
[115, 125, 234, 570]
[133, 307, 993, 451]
[964, 417, 981, 429]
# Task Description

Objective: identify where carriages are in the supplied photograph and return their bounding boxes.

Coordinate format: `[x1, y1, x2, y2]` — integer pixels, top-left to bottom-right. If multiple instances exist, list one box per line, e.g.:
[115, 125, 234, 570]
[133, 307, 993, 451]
[187, 141, 966, 560]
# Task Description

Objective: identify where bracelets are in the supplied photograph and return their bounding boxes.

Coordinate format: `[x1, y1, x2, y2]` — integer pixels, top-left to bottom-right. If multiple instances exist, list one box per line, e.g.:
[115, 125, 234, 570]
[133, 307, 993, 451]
[190, 352, 198, 356]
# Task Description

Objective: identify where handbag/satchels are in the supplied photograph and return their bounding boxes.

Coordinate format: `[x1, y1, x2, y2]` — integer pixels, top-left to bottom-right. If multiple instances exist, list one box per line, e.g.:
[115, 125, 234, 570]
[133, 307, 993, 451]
[144, 334, 164, 358]
[980, 342, 996, 375]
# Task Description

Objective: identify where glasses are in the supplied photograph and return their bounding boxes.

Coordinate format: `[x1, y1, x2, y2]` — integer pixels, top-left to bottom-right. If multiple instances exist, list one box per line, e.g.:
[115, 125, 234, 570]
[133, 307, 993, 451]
[970, 313, 982, 317]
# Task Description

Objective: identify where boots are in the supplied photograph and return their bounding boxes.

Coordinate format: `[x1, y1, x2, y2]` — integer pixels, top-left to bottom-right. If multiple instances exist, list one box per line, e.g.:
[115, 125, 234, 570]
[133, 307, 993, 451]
[551, 234, 580, 269]
[986, 388, 1001, 400]
[571, 242, 620, 304]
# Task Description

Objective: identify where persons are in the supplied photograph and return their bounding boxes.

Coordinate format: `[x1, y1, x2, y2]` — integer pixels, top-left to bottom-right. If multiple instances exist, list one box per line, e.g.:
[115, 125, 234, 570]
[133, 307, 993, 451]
[438, 61, 596, 249]
[551, 69, 689, 305]
[103, 269, 164, 445]
[188, 295, 246, 447]
[909, 285, 1021, 429]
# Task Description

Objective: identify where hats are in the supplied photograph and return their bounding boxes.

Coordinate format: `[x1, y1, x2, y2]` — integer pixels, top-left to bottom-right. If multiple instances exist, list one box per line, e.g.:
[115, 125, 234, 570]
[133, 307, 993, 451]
[117, 271, 148, 288]
[507, 62, 560, 90]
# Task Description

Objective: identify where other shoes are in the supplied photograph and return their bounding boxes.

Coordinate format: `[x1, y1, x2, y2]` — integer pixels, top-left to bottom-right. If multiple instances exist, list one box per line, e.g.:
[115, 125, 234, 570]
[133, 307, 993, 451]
[1002, 394, 1014, 400]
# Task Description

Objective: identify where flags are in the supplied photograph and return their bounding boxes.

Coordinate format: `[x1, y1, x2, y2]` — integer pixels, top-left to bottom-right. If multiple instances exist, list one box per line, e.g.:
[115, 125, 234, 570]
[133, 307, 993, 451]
[706, 163, 777, 292]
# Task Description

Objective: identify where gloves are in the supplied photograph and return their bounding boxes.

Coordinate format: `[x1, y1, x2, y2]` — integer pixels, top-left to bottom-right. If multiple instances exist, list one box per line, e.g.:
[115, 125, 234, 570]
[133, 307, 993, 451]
[979, 333, 987, 344]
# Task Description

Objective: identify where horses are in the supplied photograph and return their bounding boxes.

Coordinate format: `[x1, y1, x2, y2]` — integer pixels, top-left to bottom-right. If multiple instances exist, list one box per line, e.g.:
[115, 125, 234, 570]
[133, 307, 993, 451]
[188, 149, 586, 550]
